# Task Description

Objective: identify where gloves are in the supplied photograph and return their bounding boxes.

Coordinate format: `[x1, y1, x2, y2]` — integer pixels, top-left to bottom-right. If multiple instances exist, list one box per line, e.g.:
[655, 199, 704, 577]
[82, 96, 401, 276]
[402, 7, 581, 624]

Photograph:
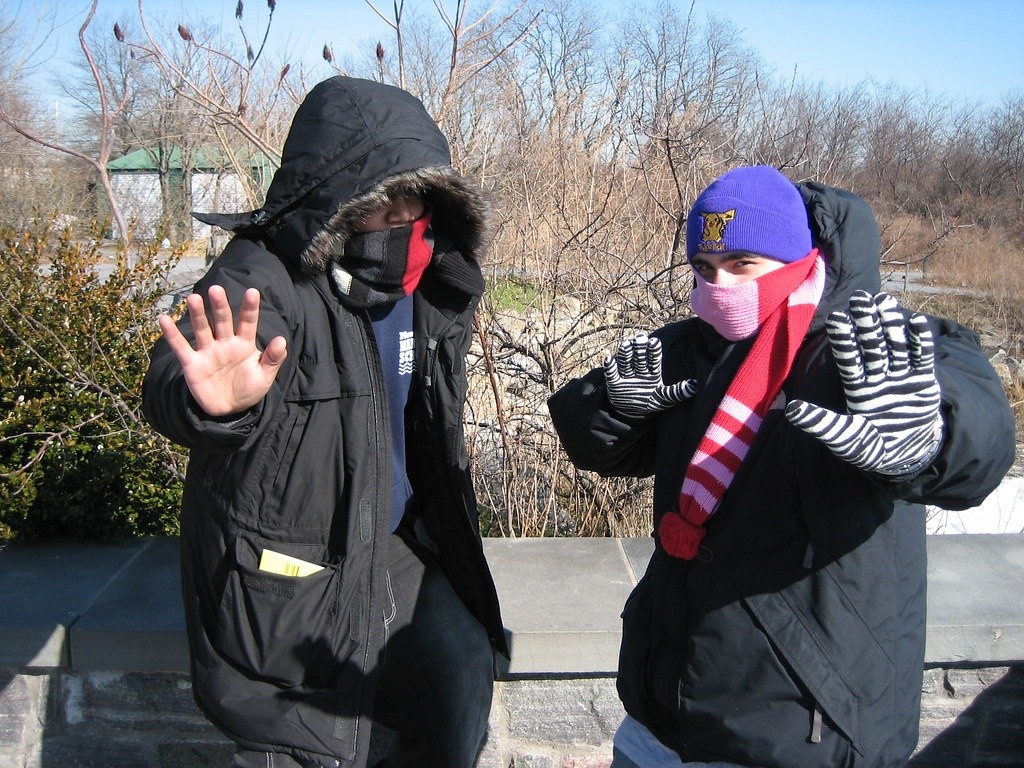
[603, 334, 698, 414]
[784, 289, 943, 475]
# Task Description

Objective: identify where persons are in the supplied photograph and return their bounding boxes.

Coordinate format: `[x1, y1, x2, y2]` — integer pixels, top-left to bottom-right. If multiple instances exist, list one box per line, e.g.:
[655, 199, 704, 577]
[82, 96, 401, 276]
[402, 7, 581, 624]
[140, 76, 514, 768]
[548, 165, 1015, 768]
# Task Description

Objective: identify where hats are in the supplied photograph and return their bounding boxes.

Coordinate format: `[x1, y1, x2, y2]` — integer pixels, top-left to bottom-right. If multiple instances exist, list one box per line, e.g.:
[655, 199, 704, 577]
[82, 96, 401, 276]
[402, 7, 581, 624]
[686, 165, 812, 262]
